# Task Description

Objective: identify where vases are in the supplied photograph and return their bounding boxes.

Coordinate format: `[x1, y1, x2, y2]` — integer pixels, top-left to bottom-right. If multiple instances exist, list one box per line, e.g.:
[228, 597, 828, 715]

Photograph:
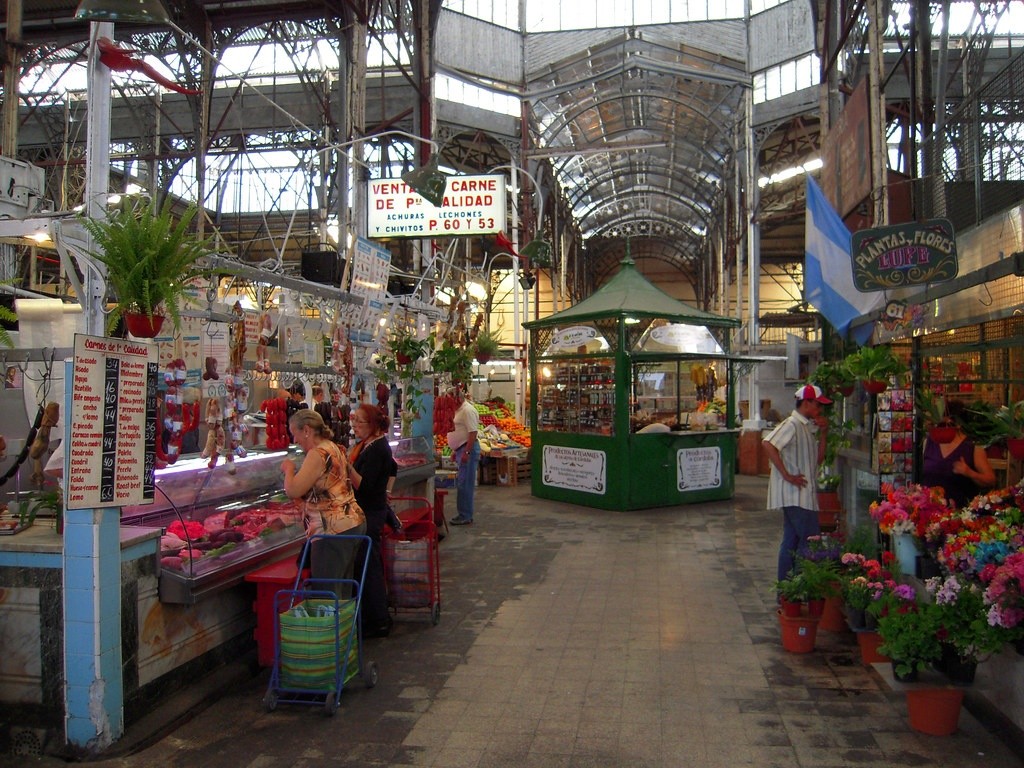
[1006, 436, 1024, 458]
[1015, 636, 1024, 656]
[776, 608, 819, 652]
[893, 532, 919, 575]
[857, 632, 890, 664]
[819, 580, 850, 633]
[905, 688, 965, 737]
[852, 606, 865, 628]
[946, 663, 976, 685]
[922, 557, 944, 583]
[891, 658, 920, 682]
[865, 612, 880, 631]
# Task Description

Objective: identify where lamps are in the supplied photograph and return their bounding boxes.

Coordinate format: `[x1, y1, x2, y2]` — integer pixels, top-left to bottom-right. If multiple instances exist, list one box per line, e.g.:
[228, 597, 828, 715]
[416, 164, 554, 298]
[71, 0, 169, 24]
[264, 130, 447, 273]
[516, 267, 537, 290]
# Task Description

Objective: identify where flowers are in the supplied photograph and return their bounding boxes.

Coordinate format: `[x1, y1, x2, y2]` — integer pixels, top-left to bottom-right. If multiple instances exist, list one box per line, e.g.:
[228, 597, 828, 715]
[968, 398, 1024, 447]
[805, 476, 1024, 639]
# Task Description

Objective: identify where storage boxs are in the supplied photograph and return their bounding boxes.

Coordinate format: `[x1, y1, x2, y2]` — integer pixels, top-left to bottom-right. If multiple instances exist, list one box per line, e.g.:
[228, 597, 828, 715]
[433, 438, 531, 490]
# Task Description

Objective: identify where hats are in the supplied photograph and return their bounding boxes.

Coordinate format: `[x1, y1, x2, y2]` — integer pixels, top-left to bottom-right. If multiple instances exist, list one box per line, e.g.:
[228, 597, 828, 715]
[794, 385, 832, 406]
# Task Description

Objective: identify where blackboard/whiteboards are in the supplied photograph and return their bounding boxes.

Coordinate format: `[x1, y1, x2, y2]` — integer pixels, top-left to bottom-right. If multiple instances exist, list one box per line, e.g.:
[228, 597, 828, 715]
[66, 333, 159, 511]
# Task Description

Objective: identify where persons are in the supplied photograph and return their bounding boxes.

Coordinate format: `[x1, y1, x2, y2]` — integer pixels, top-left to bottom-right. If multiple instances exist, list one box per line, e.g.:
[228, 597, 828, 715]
[289, 385, 323, 409]
[339, 404, 394, 637]
[447, 387, 481, 524]
[636, 422, 682, 432]
[44, 440, 62, 488]
[922, 401, 995, 577]
[762, 385, 832, 602]
[281, 410, 365, 598]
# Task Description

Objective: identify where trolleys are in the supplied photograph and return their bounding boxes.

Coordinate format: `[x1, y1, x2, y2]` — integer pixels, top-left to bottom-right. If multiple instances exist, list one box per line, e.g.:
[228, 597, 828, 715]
[379, 495, 442, 628]
[259, 532, 381, 715]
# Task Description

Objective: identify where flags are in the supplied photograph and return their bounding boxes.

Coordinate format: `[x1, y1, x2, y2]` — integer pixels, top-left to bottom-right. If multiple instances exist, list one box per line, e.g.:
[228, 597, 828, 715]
[805, 175, 892, 347]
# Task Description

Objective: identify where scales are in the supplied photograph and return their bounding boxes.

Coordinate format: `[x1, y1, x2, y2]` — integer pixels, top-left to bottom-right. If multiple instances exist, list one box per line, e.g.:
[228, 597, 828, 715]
[6, 439, 57, 518]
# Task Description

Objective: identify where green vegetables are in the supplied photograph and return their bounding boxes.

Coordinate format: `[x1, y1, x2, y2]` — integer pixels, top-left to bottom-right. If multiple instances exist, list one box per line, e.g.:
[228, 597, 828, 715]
[194, 519, 272, 555]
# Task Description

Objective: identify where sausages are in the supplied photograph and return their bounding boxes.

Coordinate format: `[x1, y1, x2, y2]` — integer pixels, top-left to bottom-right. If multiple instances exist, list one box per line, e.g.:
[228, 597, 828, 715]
[228, 302, 273, 374]
[315, 402, 351, 449]
[260, 397, 309, 450]
[333, 327, 353, 398]
[156, 357, 248, 475]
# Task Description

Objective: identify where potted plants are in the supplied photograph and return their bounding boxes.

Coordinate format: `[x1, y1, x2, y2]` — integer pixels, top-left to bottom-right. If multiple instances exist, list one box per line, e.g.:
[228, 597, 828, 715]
[70, 189, 243, 339]
[15, 483, 64, 534]
[822, 360, 855, 396]
[798, 552, 846, 616]
[812, 471, 843, 526]
[472, 329, 504, 363]
[771, 568, 808, 617]
[913, 390, 959, 444]
[847, 347, 904, 394]
[376, 334, 435, 413]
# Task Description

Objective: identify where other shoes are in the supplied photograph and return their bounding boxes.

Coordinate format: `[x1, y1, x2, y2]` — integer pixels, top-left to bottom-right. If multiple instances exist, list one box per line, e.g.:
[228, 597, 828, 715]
[376, 619, 391, 636]
[451, 514, 472, 525]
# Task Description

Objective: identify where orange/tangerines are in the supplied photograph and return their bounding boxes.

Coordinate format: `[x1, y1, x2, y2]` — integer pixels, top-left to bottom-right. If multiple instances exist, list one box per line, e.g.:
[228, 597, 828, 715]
[498, 419, 530, 447]
[437, 434, 448, 445]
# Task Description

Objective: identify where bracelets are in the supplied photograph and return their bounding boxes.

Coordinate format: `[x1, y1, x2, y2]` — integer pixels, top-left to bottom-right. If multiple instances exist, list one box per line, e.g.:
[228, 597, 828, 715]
[464, 451, 471, 454]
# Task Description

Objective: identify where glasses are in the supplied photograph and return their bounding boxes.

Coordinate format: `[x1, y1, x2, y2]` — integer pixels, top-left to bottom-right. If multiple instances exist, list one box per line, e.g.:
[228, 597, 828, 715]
[352, 415, 368, 424]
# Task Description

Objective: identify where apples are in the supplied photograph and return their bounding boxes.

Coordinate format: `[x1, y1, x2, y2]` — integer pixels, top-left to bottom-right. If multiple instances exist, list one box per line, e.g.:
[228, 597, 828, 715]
[443, 447, 452, 456]
[474, 404, 503, 430]
